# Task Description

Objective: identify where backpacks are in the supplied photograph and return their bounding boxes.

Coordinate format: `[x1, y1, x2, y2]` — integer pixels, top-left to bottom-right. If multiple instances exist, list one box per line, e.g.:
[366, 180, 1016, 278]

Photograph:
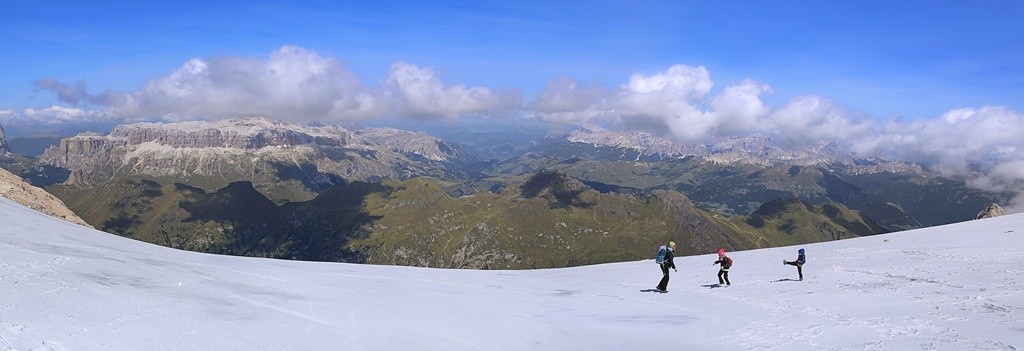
[724, 258, 732, 266]
[656, 246, 673, 264]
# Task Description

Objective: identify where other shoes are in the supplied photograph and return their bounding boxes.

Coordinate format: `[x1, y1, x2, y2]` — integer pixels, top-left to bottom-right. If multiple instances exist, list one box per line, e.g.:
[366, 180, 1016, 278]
[657, 286, 667, 293]
[783, 260, 786, 265]
[799, 277, 803, 281]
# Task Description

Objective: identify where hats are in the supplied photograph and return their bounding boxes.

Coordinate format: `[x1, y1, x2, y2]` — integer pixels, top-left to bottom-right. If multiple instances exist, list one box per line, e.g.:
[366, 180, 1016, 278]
[799, 249, 804, 254]
[668, 241, 675, 247]
[719, 249, 724, 256]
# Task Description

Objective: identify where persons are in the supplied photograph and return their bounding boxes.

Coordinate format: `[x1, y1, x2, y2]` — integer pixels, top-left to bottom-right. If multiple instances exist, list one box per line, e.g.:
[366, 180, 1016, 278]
[712, 249, 731, 286]
[783, 248, 805, 281]
[656, 241, 677, 293]
